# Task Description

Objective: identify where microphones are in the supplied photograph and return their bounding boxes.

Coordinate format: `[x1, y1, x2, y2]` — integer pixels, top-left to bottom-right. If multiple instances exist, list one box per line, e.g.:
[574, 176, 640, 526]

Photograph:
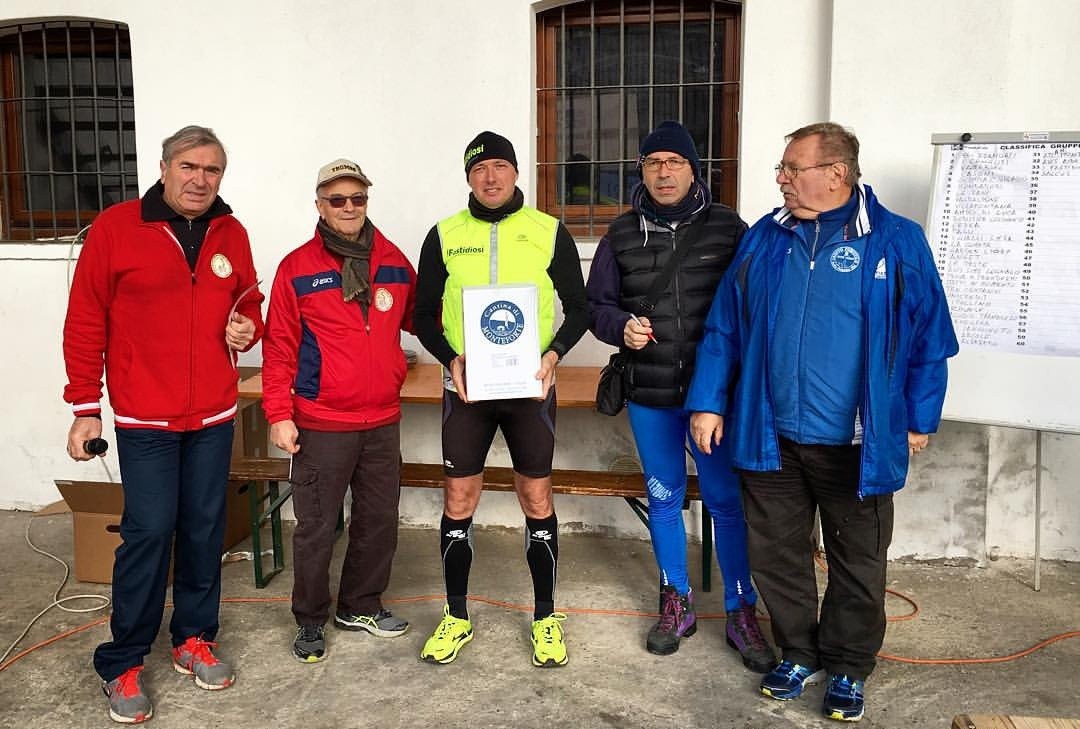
[84, 438, 108, 455]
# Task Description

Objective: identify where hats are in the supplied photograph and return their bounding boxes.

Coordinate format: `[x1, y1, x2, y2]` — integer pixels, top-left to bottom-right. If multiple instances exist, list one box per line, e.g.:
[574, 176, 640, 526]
[316, 159, 372, 194]
[464, 131, 518, 181]
[640, 120, 700, 178]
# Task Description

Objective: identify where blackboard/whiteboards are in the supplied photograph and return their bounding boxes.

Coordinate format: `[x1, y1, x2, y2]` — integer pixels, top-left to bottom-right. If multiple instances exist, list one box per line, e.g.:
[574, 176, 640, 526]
[924, 133, 1076, 438]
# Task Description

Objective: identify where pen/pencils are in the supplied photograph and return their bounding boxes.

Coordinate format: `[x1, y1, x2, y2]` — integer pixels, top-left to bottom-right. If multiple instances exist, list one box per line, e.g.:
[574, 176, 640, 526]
[631, 314, 657, 345]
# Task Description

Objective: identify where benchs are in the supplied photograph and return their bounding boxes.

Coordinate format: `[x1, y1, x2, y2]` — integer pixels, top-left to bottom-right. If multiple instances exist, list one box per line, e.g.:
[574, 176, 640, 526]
[226, 458, 701, 500]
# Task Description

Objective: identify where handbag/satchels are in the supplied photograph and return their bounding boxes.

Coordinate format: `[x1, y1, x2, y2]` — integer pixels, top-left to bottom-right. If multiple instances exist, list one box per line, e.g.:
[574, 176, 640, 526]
[595, 353, 633, 416]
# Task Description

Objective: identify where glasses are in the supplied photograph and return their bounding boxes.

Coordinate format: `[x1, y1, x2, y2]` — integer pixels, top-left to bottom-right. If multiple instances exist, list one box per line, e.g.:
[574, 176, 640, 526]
[322, 192, 368, 208]
[642, 158, 689, 172]
[774, 163, 845, 180]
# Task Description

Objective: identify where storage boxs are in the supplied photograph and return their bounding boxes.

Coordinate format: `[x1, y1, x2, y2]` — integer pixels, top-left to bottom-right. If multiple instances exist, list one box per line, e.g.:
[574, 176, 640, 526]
[30, 479, 265, 588]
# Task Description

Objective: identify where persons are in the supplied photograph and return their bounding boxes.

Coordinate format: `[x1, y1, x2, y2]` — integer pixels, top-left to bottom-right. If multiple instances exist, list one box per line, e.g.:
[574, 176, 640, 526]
[63, 126, 265, 723]
[686, 123, 958, 722]
[587, 120, 779, 674]
[261, 159, 444, 663]
[419, 131, 588, 667]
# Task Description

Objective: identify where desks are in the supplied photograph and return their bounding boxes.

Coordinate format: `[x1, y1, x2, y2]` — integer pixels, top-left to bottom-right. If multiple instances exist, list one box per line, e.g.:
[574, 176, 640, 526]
[235, 365, 716, 592]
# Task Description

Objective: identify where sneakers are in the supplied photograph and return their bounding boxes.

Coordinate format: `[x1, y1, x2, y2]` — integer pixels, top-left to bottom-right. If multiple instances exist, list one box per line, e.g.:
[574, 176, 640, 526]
[292, 624, 330, 663]
[647, 585, 696, 655]
[823, 673, 864, 721]
[531, 612, 569, 667]
[726, 606, 779, 671]
[172, 632, 235, 691]
[420, 604, 473, 664]
[759, 660, 827, 700]
[102, 666, 153, 723]
[334, 609, 408, 638]
